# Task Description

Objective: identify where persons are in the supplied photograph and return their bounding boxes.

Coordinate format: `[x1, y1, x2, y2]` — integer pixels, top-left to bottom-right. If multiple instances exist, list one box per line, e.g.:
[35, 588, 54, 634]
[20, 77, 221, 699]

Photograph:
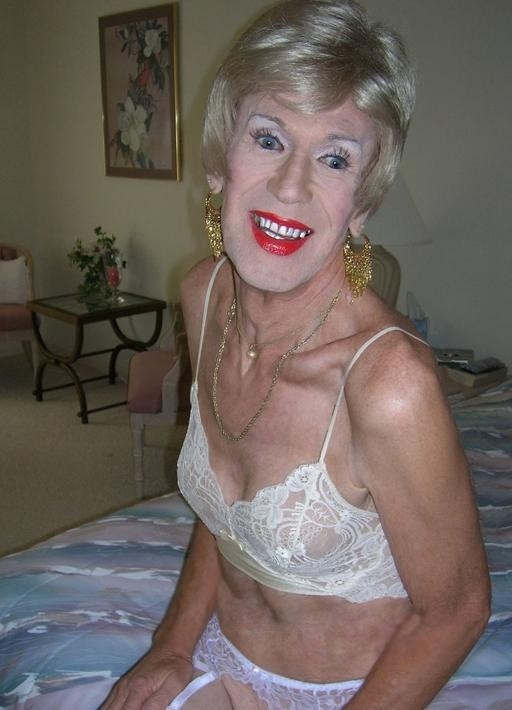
[97, 2, 493, 710]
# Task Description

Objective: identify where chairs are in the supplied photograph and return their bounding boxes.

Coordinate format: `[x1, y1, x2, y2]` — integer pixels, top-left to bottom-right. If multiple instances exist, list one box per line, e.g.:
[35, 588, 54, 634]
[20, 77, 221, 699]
[0, 242, 42, 388]
[125, 300, 189, 499]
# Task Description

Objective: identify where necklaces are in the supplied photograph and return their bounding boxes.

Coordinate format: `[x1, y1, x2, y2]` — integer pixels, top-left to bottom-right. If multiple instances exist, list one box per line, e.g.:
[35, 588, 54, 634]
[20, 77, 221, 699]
[210, 285, 344, 442]
[232, 308, 327, 360]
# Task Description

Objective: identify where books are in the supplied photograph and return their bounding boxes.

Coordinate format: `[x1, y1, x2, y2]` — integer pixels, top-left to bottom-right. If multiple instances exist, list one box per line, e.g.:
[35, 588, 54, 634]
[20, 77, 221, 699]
[447, 364, 510, 388]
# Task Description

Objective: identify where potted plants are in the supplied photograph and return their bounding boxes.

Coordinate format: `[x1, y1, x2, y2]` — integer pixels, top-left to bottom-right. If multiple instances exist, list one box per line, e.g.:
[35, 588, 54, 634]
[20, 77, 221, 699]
[66, 225, 128, 303]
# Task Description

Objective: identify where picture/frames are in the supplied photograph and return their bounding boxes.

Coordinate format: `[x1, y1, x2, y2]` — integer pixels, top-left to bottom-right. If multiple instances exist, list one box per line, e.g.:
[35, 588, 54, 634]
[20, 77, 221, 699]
[96, 3, 180, 182]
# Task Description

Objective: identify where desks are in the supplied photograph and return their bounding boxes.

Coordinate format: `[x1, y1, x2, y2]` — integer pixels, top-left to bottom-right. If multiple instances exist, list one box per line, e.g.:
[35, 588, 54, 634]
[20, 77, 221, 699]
[25, 286, 166, 425]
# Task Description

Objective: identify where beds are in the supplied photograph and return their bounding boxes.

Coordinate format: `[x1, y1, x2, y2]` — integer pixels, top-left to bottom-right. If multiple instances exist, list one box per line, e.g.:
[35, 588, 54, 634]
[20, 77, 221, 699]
[2, 388, 510, 710]
